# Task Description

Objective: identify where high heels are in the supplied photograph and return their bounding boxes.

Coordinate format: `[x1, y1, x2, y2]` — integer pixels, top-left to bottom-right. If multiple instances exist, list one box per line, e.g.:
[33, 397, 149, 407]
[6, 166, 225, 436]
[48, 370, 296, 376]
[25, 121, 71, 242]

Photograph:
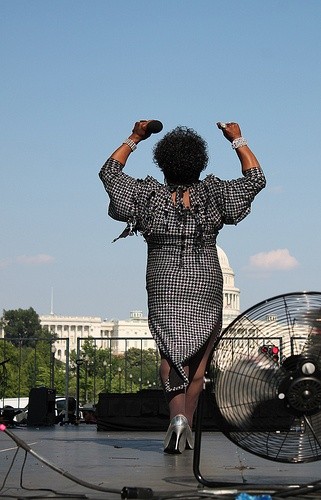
[164, 414, 194, 454]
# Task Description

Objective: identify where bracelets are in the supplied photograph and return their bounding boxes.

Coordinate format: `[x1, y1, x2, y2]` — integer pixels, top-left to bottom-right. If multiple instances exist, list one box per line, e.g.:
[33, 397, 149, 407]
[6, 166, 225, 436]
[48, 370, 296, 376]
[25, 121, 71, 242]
[123, 139, 137, 152]
[232, 137, 248, 149]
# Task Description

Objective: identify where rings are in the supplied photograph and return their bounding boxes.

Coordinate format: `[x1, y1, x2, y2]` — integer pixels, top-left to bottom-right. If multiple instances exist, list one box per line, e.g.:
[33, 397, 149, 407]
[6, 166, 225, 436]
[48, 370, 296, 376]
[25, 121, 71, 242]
[225, 122, 231, 124]
[220, 123, 226, 128]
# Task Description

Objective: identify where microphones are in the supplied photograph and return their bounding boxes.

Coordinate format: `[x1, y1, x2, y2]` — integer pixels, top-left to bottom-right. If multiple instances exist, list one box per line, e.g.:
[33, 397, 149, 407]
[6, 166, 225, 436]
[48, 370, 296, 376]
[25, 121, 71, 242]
[132, 120, 163, 133]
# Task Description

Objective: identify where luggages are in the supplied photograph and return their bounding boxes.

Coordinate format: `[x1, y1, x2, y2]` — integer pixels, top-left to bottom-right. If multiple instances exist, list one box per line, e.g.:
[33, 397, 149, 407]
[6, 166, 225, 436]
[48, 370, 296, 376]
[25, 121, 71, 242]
[26, 386, 57, 427]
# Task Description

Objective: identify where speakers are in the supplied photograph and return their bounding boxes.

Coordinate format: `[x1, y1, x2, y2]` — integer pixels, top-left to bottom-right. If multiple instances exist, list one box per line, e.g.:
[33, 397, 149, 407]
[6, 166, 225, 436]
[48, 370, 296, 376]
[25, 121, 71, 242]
[197, 392, 293, 433]
[27, 388, 56, 425]
[97, 392, 197, 432]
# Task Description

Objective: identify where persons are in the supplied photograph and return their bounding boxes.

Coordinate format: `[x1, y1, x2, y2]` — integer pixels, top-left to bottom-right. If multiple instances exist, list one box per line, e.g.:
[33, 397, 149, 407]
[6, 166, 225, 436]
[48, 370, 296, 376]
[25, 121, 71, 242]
[99, 119, 267, 454]
